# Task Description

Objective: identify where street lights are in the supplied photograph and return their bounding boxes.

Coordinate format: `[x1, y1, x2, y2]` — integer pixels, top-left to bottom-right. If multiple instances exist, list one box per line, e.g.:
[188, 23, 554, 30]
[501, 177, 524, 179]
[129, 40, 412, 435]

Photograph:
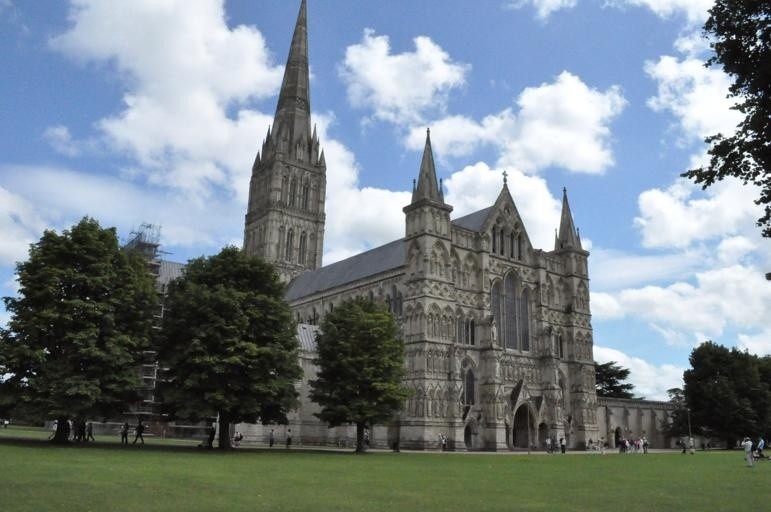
[686, 408, 695, 455]
[526, 392, 530, 456]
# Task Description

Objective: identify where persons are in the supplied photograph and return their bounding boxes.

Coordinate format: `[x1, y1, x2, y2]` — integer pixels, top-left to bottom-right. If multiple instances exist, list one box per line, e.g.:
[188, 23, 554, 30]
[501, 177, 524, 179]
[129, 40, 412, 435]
[741, 436, 764, 467]
[546, 437, 567, 454]
[440, 435, 447, 451]
[122, 421, 147, 446]
[285, 428, 293, 449]
[588, 436, 605, 452]
[621, 436, 649, 454]
[50, 416, 95, 444]
[268, 429, 275, 448]
[233, 430, 244, 445]
[679, 435, 696, 454]
